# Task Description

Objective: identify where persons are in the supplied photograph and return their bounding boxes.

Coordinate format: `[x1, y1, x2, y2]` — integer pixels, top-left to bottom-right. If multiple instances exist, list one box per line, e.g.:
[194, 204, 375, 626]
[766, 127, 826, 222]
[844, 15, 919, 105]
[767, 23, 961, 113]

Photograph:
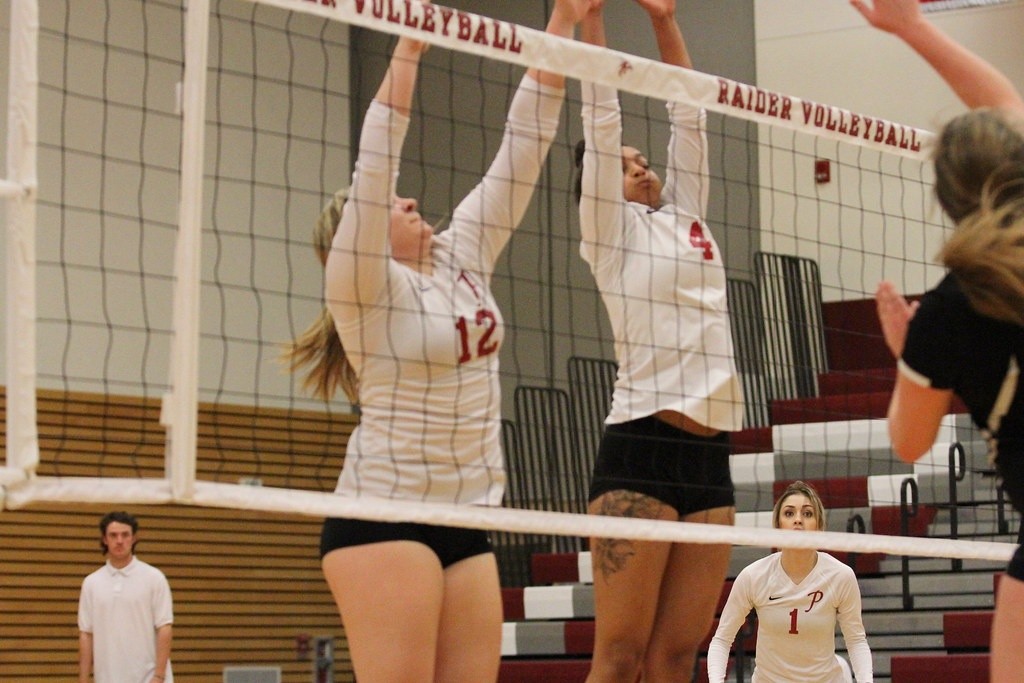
[708, 485, 874, 683]
[78, 510, 174, 683]
[851, 0, 1024, 683]
[579, 0, 743, 683]
[284, 0, 592, 683]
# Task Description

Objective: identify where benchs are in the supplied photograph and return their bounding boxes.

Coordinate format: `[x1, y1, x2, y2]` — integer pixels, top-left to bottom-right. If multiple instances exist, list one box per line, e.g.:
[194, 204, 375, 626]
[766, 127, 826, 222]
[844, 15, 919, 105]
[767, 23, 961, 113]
[492, 292, 1020, 683]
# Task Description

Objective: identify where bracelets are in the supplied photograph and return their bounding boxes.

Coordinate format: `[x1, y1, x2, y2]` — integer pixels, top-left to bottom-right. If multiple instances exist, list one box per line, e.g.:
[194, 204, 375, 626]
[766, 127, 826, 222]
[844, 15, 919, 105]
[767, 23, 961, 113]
[154, 675, 164, 680]
